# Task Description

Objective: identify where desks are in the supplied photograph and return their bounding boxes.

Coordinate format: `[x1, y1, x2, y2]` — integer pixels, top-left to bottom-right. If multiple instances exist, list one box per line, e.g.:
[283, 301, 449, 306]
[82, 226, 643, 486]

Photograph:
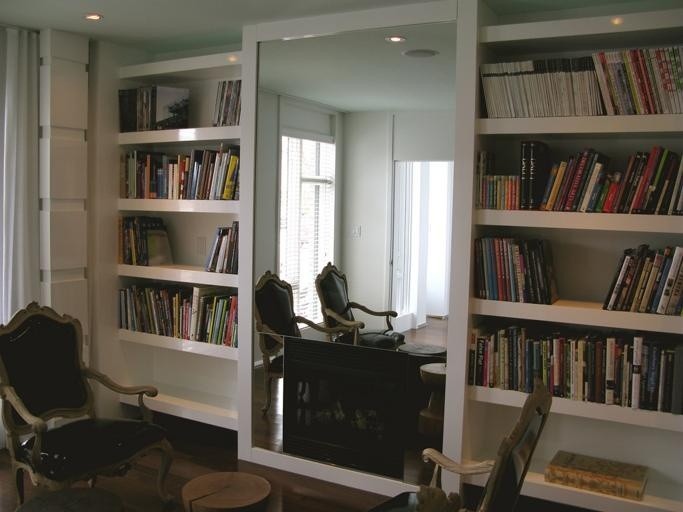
[396, 341, 447, 362]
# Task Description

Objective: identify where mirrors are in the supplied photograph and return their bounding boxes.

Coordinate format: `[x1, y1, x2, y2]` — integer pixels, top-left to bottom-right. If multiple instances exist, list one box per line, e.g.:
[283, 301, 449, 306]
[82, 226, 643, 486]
[249, 8, 464, 490]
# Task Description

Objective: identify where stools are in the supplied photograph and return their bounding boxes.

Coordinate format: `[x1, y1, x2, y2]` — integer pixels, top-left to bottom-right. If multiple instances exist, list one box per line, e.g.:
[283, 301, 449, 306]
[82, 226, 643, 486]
[180, 470, 272, 511]
[417, 361, 446, 439]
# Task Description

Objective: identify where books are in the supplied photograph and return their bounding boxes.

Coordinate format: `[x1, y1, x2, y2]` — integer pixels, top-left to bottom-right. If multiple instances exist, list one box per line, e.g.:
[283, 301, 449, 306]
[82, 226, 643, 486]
[118, 86, 189, 133]
[603, 245, 682, 316]
[119, 284, 238, 348]
[475, 141, 682, 216]
[540, 449, 651, 504]
[205, 221, 239, 275]
[212, 80, 240, 127]
[468, 319, 682, 414]
[120, 142, 239, 200]
[119, 213, 174, 266]
[479, 44, 683, 118]
[115, 79, 241, 350]
[474, 235, 560, 305]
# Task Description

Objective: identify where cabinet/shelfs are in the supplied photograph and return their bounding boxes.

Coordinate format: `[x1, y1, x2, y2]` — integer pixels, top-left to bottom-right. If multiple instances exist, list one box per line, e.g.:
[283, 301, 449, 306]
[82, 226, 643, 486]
[461, 9, 683, 512]
[110, 49, 239, 434]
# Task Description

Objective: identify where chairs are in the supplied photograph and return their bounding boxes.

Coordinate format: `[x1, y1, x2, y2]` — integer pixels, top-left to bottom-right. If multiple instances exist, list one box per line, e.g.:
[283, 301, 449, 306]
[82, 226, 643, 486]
[365, 383, 553, 511]
[0, 299, 177, 510]
[254, 253, 406, 418]
